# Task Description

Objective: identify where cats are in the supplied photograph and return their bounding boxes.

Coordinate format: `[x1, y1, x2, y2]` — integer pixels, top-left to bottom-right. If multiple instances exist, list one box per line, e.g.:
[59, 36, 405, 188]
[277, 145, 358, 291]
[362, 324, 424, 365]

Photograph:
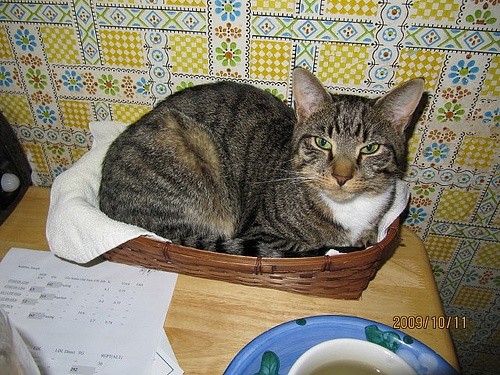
[99, 67, 425, 258]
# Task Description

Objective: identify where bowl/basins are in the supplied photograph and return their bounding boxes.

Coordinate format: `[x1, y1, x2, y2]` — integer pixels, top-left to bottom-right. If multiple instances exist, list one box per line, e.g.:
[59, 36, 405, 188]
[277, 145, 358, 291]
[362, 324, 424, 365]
[287, 338, 419, 375]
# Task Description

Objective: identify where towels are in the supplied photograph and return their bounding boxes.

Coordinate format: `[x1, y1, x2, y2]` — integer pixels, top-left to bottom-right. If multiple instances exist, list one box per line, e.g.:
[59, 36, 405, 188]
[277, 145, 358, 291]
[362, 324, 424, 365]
[45, 119, 413, 266]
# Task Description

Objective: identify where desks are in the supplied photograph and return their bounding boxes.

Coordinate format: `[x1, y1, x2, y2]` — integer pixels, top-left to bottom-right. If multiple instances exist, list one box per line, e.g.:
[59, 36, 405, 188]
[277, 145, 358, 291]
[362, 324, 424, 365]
[1, 187, 460, 375]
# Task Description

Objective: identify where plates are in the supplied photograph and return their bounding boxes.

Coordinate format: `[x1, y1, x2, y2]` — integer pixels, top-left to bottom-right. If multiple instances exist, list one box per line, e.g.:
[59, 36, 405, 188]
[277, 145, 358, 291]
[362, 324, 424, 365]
[222, 314, 461, 374]
